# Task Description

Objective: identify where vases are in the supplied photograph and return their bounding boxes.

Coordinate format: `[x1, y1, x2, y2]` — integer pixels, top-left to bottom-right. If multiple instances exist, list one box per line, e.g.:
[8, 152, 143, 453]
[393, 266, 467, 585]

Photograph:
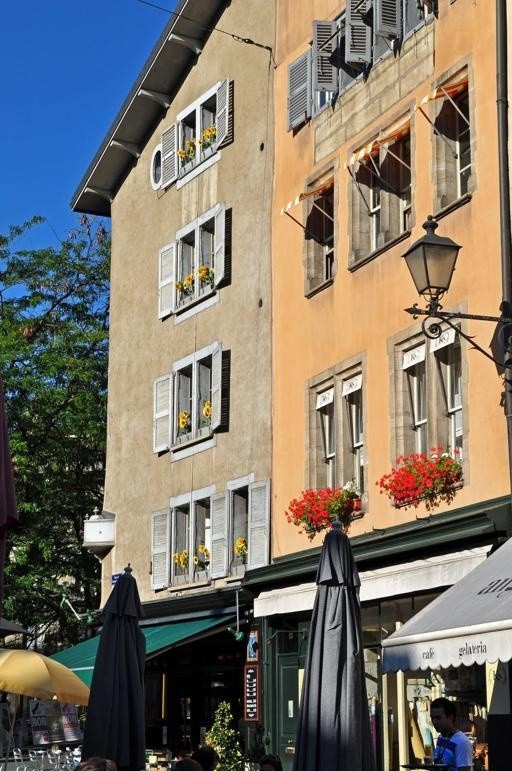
[242, 556, 248, 564]
[352, 498, 361, 511]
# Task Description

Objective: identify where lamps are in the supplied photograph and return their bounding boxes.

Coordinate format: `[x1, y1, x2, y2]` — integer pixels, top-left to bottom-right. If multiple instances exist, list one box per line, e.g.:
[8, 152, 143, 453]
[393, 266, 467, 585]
[228, 589, 245, 642]
[265, 628, 308, 645]
[401, 215, 512, 490]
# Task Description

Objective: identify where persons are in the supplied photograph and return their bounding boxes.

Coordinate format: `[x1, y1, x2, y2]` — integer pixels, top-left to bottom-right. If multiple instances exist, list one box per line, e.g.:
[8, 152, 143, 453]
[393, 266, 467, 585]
[41, 732, 49, 744]
[192, 746, 219, 771]
[429, 697, 473, 771]
[259, 752, 283, 771]
[73, 757, 119, 770]
[175, 758, 202, 771]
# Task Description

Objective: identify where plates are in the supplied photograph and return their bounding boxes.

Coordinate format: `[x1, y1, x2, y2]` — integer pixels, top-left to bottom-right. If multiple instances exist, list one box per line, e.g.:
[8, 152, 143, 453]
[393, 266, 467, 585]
[402, 765, 453, 769]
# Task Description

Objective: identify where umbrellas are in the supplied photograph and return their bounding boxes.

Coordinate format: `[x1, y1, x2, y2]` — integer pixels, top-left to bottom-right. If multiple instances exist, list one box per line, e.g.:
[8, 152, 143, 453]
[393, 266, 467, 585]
[81, 562, 147, 771]
[0, 616, 36, 641]
[0, 647, 90, 707]
[293, 521, 377, 771]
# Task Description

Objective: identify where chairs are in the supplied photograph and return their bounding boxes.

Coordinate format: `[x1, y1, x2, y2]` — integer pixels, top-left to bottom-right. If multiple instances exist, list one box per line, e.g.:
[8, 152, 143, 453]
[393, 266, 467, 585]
[13, 745, 83, 771]
[144, 755, 158, 771]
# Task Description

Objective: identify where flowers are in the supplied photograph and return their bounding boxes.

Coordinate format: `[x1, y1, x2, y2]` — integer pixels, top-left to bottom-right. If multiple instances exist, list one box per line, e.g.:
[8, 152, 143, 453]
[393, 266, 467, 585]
[232, 537, 248, 555]
[285, 480, 360, 544]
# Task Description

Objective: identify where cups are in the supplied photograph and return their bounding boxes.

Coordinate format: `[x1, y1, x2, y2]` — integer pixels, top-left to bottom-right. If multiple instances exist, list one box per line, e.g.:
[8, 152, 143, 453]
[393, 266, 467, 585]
[424, 757, 433, 764]
[409, 758, 421, 766]
[423, 746, 432, 756]
